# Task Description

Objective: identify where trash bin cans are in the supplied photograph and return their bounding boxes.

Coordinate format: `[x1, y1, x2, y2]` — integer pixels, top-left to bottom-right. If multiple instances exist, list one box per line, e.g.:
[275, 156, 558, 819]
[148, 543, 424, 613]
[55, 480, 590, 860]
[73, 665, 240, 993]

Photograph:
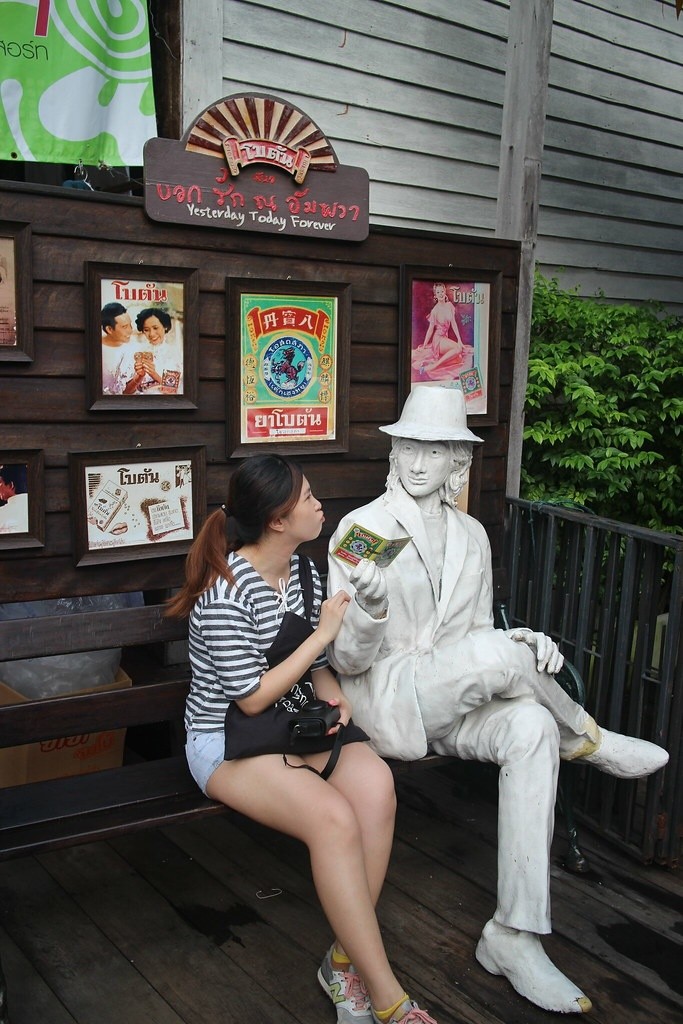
[0, 664, 133, 790]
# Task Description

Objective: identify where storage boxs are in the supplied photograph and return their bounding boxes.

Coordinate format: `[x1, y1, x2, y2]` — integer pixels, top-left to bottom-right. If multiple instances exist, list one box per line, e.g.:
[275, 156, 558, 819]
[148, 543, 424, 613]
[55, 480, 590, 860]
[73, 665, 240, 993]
[0, 666, 133, 790]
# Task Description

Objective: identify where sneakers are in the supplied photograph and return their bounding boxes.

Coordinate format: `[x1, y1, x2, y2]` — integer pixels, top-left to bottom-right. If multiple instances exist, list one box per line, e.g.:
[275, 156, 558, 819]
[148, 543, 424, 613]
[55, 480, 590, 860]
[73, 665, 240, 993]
[371, 992, 437, 1024]
[317, 944, 373, 1024]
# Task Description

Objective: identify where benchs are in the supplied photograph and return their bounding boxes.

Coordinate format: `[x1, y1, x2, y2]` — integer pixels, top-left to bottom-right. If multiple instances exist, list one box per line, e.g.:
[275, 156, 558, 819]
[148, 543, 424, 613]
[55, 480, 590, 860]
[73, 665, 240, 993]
[0, 599, 595, 876]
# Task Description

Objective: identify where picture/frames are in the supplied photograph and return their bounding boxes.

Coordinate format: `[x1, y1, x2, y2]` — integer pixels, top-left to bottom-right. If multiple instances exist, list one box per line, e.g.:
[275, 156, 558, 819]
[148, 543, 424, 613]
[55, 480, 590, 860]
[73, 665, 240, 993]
[397, 263, 504, 426]
[224, 275, 352, 459]
[0, 219, 36, 363]
[84, 260, 201, 411]
[67, 445, 210, 569]
[0, 447, 46, 551]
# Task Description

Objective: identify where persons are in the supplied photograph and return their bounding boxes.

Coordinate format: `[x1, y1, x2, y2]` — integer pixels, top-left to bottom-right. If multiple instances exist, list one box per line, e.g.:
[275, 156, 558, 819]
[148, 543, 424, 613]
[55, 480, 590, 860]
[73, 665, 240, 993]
[102, 302, 184, 395]
[163, 454, 437, 1024]
[413, 282, 475, 382]
[330, 389, 669, 1012]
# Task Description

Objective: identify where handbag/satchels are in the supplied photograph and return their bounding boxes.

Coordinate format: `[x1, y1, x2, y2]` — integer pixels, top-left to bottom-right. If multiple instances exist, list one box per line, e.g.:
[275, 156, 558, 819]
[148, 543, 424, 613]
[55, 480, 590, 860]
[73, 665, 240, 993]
[222, 549, 370, 760]
